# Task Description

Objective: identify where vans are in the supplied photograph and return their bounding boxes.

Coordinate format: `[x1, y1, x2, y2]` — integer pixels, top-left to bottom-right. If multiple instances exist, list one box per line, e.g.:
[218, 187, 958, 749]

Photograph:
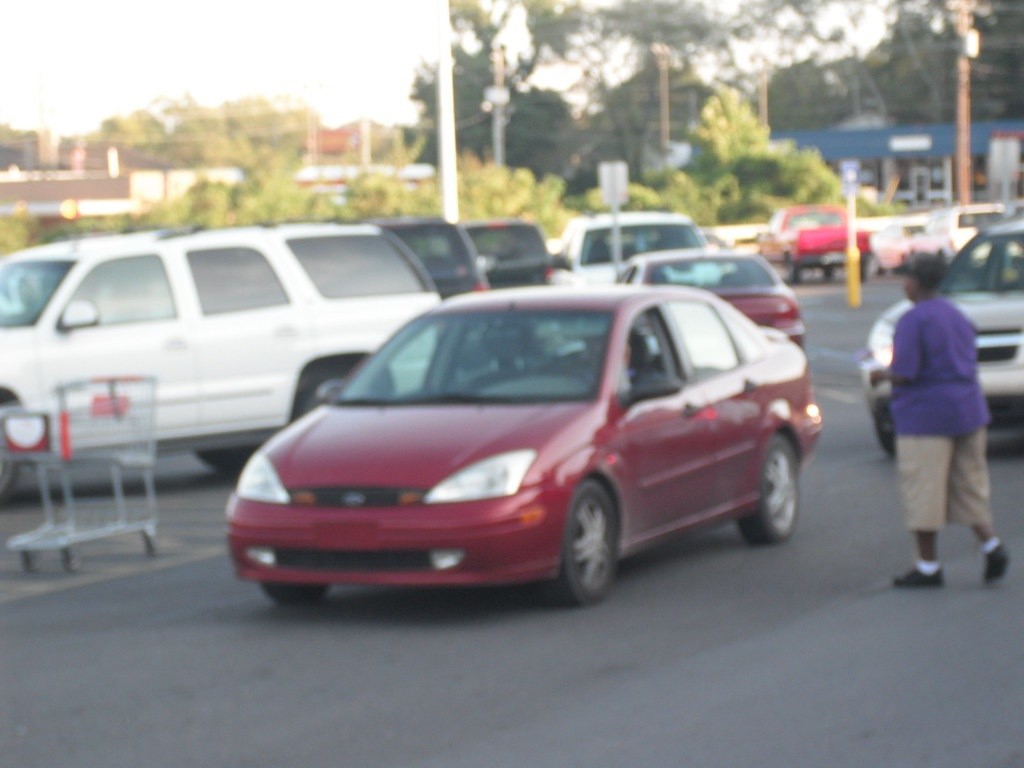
[2, 219, 440, 495]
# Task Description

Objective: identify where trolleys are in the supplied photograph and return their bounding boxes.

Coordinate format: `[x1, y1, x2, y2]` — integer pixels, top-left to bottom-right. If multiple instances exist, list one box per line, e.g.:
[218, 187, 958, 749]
[2, 375, 163, 581]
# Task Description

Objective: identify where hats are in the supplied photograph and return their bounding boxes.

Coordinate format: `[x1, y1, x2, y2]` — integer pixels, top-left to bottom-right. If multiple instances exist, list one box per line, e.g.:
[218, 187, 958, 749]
[906, 251, 944, 279]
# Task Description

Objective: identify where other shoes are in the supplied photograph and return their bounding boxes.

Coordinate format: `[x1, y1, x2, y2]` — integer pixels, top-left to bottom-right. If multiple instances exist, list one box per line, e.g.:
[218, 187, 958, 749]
[893, 568, 942, 586]
[985, 545, 1009, 580]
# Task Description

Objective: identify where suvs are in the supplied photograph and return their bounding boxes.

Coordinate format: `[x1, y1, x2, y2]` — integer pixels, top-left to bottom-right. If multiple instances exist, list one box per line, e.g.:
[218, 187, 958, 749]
[861, 217, 1024, 456]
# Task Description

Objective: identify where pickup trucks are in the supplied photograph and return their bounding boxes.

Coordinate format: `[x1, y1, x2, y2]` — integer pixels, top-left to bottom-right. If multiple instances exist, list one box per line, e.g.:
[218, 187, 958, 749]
[772, 193, 873, 285]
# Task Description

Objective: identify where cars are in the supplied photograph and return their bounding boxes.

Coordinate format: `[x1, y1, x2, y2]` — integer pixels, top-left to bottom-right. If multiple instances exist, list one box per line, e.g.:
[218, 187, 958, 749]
[219, 285, 824, 608]
[384, 201, 808, 346]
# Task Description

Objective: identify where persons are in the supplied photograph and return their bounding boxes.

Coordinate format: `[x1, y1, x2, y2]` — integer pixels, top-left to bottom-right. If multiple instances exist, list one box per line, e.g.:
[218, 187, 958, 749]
[864, 246, 1014, 593]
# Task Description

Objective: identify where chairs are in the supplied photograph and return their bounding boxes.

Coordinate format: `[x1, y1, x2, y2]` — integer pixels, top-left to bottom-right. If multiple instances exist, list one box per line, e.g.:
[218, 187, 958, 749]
[461, 322, 548, 398]
